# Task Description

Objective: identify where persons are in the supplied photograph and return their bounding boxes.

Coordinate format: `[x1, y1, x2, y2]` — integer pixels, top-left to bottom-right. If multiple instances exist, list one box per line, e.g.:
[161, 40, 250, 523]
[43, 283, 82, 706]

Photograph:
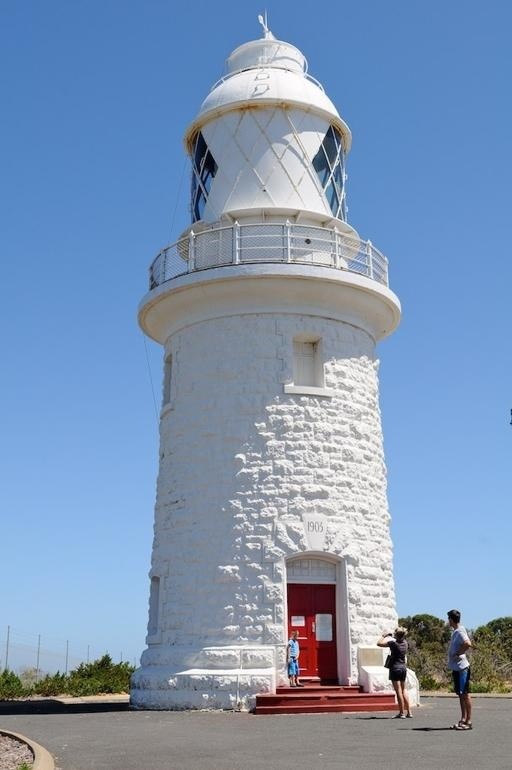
[377, 626, 413, 718]
[287, 630, 304, 687]
[447, 609, 472, 730]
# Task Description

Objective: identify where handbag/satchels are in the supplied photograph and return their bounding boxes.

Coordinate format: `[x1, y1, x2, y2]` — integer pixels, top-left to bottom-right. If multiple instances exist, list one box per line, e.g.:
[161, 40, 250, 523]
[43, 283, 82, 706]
[384, 655, 392, 668]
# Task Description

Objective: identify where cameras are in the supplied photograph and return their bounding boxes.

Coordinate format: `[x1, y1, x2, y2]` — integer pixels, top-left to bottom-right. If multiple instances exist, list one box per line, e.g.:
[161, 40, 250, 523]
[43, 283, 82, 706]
[388, 633, 392, 636]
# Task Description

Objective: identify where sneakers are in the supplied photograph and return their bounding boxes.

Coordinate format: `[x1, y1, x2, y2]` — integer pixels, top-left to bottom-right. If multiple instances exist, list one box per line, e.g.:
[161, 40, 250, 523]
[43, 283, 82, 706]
[406, 713, 412, 718]
[297, 683, 304, 687]
[290, 683, 296, 687]
[394, 713, 405, 719]
[457, 721, 472, 730]
[450, 720, 465, 729]
